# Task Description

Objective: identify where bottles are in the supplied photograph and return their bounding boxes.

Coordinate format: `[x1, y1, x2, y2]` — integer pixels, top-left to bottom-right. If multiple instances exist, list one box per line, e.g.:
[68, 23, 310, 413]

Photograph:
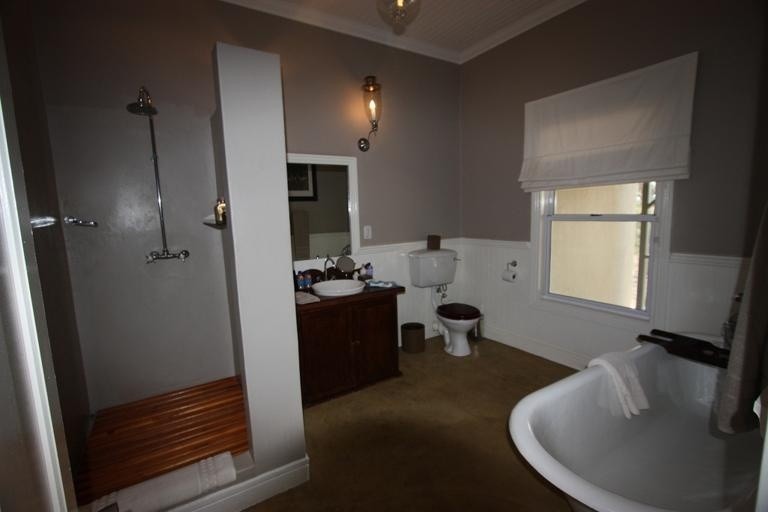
[296, 270, 312, 290]
[367, 262, 373, 279]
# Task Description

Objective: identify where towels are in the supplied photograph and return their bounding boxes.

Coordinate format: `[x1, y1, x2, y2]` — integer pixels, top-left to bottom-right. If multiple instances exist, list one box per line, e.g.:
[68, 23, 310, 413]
[587, 351, 650, 420]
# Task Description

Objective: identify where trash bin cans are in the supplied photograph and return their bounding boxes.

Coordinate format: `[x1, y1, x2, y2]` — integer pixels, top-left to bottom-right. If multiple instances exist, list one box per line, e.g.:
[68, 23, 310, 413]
[401, 322, 425, 354]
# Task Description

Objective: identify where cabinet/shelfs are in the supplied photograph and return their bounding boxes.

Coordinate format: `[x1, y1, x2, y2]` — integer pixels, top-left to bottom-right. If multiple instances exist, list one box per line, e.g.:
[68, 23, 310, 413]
[295, 295, 398, 409]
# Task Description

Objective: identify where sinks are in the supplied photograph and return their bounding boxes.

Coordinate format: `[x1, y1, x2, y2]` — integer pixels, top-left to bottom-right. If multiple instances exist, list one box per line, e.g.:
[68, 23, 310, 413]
[312, 279, 365, 297]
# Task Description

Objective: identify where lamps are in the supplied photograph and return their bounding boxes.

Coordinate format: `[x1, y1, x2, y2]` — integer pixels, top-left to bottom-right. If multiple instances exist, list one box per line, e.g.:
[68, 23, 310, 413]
[359, 74, 383, 151]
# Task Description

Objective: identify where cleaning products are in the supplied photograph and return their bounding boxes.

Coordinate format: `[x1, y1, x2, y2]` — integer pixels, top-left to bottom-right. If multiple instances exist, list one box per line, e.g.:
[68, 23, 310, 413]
[213, 197, 226, 223]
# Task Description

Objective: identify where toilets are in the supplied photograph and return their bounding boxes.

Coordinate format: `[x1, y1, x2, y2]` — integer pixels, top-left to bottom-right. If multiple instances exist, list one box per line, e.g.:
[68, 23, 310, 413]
[410, 248, 480, 357]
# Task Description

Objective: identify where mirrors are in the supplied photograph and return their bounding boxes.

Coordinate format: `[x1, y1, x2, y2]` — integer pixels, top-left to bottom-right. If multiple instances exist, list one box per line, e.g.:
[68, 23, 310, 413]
[286, 152, 363, 275]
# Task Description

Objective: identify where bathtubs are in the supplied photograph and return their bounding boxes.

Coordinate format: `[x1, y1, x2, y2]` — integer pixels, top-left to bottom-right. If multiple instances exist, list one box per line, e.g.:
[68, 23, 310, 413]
[509, 331, 757, 512]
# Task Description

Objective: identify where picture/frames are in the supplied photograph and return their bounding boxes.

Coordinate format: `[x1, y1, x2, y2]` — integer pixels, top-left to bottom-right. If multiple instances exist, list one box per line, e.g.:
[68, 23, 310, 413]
[287, 163, 318, 201]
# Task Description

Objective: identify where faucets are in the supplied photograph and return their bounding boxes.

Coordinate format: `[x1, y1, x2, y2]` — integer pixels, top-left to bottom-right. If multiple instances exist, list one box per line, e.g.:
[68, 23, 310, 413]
[324, 257, 335, 279]
